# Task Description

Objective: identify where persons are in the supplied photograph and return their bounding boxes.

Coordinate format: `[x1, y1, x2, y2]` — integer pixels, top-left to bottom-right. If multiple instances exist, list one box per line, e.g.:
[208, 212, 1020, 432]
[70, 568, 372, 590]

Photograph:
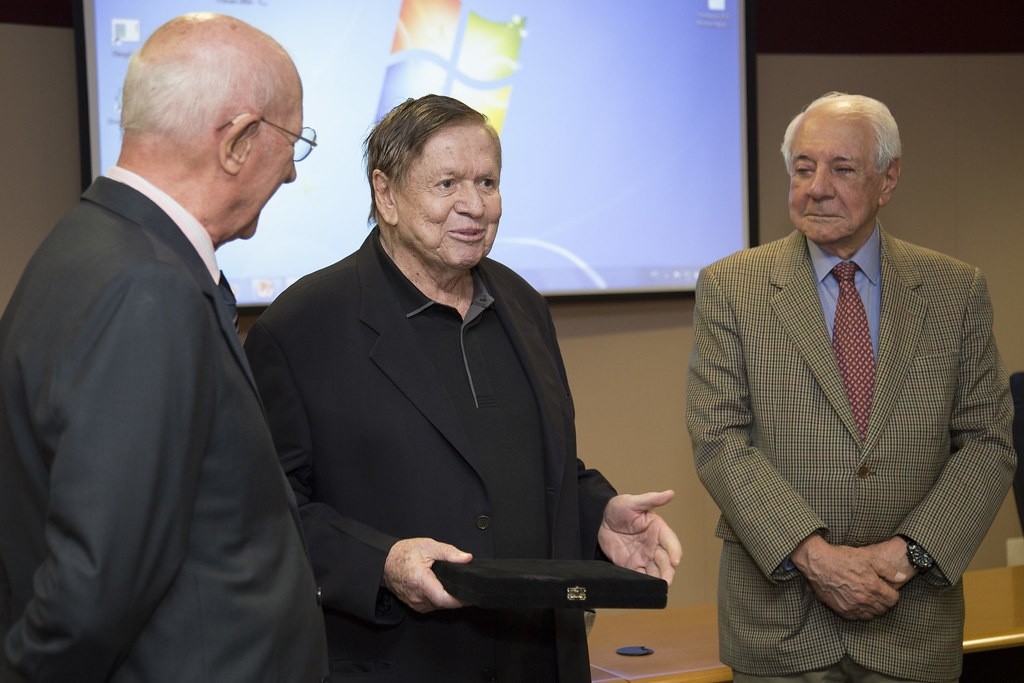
[686, 91, 1017, 683]
[242, 95, 683, 683]
[0, 11, 330, 683]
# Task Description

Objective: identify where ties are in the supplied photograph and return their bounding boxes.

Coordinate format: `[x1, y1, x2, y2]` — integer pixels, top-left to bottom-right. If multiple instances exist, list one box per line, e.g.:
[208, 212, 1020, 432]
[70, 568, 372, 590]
[830, 261, 878, 439]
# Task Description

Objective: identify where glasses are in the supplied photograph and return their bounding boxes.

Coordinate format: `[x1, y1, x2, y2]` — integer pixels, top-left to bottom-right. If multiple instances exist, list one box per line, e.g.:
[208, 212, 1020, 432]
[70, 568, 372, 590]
[214, 112, 317, 164]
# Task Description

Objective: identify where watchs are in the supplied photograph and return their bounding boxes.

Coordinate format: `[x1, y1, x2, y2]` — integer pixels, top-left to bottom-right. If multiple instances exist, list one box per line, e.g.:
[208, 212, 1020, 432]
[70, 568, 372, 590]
[899, 534, 933, 575]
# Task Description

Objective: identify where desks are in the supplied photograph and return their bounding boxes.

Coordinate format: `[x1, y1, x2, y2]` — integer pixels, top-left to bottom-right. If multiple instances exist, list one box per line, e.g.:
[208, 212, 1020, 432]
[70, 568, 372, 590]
[587, 559, 1024, 683]
